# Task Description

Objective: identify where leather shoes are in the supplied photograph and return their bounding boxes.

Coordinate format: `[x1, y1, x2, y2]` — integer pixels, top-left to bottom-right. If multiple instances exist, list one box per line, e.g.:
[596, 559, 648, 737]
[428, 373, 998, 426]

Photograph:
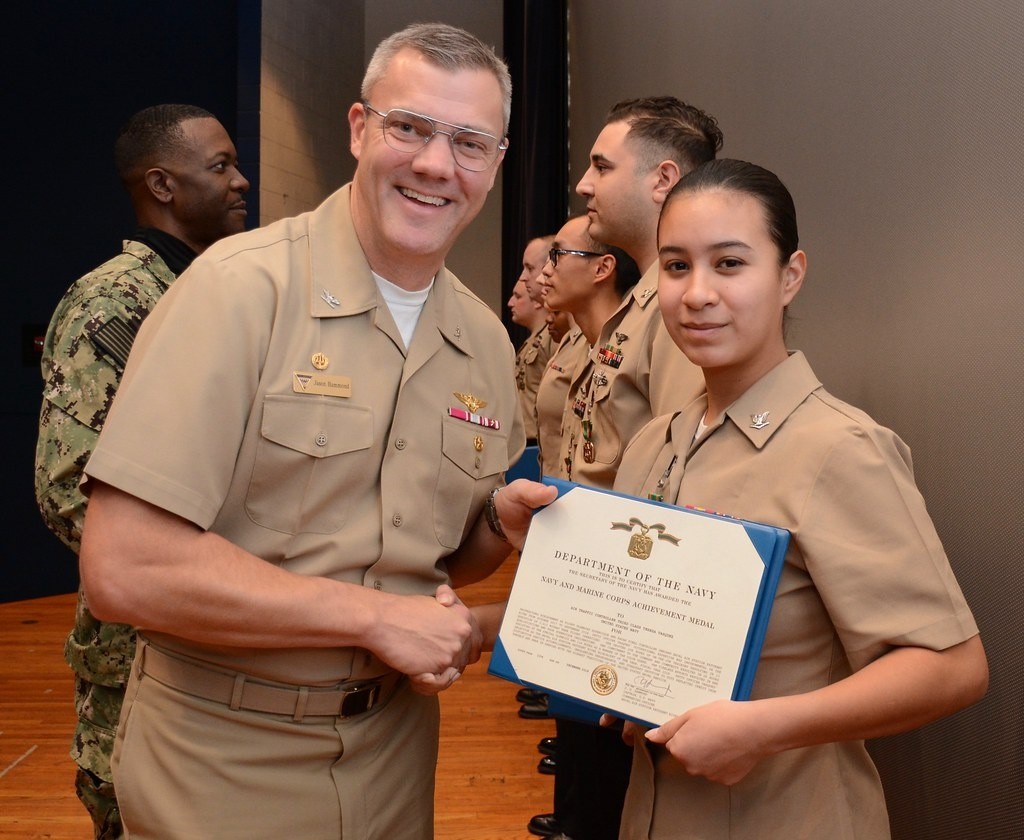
[538, 737, 559, 774]
[528, 813, 572, 840]
[514, 688, 553, 719]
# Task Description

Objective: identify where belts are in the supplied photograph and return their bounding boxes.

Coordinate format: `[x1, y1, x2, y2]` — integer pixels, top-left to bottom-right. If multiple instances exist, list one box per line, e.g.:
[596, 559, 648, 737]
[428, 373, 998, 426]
[136, 642, 381, 717]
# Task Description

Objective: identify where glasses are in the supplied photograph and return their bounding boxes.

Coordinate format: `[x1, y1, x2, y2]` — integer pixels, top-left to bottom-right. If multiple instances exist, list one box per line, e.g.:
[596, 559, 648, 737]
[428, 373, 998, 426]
[550, 248, 604, 267]
[367, 100, 509, 173]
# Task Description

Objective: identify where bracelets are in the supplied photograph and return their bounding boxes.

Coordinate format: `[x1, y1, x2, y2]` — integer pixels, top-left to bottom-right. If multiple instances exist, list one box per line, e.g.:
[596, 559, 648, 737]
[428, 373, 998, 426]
[485, 486, 509, 541]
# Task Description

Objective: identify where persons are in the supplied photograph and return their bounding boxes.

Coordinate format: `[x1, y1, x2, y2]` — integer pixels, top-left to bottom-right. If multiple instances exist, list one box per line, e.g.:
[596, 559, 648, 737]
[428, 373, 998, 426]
[509, 96, 721, 840]
[34, 103, 252, 840]
[598, 158, 989, 840]
[77, 25, 558, 840]
